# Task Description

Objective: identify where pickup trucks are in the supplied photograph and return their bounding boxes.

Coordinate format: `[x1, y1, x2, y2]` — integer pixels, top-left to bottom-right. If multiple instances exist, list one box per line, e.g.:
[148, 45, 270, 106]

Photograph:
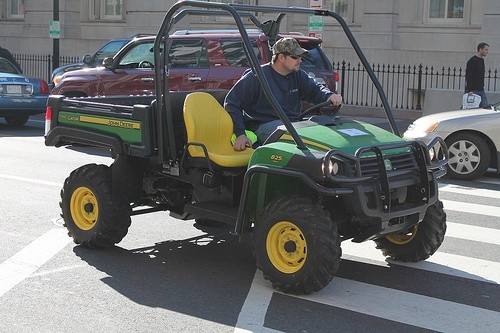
[44, 0, 447, 295]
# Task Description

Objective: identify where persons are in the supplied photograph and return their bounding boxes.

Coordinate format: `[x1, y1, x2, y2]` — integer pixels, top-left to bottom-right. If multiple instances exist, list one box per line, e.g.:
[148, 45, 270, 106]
[460, 42, 490, 110]
[223, 37, 342, 152]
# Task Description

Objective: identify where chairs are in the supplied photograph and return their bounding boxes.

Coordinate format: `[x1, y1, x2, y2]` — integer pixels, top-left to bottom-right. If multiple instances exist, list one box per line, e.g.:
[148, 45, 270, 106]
[183, 91, 255, 168]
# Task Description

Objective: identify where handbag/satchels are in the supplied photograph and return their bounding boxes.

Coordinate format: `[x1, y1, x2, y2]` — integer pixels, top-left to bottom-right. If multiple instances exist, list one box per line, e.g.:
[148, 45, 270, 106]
[462, 92, 481, 109]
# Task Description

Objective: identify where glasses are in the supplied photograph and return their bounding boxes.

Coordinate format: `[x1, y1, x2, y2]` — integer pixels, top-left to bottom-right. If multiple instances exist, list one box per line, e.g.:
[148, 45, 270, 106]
[282, 53, 305, 59]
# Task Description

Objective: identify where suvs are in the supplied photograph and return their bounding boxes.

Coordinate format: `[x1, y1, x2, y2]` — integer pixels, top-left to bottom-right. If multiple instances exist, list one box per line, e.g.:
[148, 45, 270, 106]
[50, 30, 339, 96]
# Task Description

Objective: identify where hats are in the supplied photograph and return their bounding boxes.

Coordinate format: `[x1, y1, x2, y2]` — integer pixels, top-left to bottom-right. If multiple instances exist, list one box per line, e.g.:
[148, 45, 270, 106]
[272, 37, 309, 55]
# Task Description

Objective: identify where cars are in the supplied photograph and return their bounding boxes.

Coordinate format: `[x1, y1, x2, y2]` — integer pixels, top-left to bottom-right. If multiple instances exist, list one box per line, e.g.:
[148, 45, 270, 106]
[0, 57, 50, 126]
[403, 101, 500, 181]
